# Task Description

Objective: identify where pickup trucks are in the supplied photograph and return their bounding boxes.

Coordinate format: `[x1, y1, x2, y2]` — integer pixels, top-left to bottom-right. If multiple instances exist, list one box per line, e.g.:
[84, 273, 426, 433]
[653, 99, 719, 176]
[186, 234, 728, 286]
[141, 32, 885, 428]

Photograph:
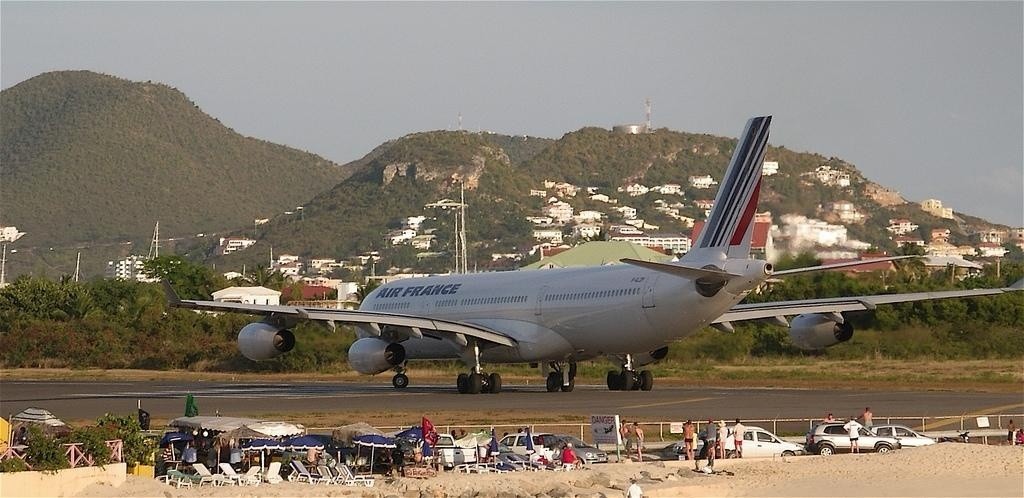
[432, 433, 476, 470]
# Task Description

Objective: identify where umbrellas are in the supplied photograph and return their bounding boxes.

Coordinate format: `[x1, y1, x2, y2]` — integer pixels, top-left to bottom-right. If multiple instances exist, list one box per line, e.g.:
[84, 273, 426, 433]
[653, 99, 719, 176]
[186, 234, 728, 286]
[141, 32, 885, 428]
[11, 406, 67, 426]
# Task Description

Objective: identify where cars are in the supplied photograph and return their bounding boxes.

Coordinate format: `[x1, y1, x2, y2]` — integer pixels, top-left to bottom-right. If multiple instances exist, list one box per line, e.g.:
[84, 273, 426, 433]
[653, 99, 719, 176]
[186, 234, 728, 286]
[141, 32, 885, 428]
[550, 436, 609, 466]
[16, 420, 77, 441]
[711, 427, 808, 459]
[669, 425, 734, 459]
[494, 432, 560, 463]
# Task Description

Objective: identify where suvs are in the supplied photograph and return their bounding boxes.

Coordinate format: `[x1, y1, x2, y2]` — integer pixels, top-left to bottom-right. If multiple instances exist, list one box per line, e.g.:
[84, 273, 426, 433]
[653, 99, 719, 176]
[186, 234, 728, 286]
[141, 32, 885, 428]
[805, 422, 904, 455]
[869, 423, 938, 447]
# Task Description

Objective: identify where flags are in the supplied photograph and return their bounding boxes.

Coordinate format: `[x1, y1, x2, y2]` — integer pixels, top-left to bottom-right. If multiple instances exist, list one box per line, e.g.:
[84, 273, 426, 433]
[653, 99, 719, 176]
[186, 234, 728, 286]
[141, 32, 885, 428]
[137, 407, 150, 429]
[184, 391, 198, 417]
[422, 417, 439, 449]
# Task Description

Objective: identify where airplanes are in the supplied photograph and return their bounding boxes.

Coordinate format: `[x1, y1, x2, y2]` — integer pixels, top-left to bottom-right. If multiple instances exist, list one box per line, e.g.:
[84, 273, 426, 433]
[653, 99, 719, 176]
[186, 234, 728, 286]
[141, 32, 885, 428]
[162, 114, 1023, 397]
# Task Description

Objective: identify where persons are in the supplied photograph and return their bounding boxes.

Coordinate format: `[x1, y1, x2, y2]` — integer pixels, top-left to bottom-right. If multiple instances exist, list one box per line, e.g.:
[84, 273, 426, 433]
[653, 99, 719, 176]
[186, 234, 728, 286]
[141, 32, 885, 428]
[716, 419, 728, 459]
[862, 405, 873, 431]
[732, 417, 746, 459]
[625, 480, 644, 497]
[683, 419, 696, 460]
[632, 421, 644, 462]
[618, 420, 634, 458]
[1015, 427, 1024, 445]
[1006, 418, 1015, 444]
[697, 417, 717, 473]
[560, 446, 566, 461]
[529, 449, 548, 465]
[822, 413, 835, 432]
[843, 416, 862, 452]
[560, 442, 581, 470]
[19, 426, 26, 444]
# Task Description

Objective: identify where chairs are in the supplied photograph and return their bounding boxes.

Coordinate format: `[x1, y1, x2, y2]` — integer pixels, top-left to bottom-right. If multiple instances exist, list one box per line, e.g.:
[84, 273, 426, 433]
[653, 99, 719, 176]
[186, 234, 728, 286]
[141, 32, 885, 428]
[452, 444, 575, 473]
[159, 460, 376, 490]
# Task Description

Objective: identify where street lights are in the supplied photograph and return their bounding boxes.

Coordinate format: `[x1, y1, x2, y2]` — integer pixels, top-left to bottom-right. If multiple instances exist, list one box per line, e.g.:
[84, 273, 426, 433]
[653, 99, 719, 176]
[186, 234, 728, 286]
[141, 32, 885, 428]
[0, 224, 26, 289]
[422, 197, 471, 275]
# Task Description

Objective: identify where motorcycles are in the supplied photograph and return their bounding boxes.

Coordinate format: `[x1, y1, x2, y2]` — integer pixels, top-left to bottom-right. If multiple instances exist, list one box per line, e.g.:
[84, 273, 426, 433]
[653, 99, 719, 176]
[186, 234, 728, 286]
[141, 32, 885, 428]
[937, 428, 971, 445]
